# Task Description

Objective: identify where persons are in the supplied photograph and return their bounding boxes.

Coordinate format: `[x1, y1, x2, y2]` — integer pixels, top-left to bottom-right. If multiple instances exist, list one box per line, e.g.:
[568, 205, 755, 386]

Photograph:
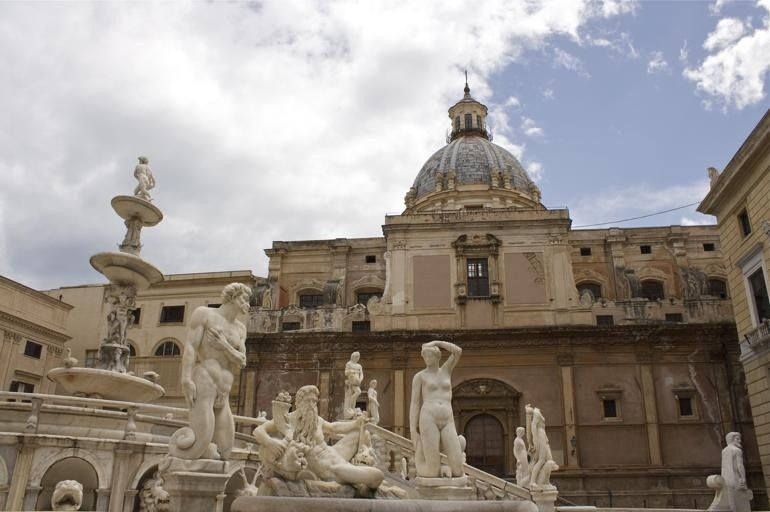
[98, 152, 155, 375]
[527, 406, 553, 491]
[344, 350, 363, 410]
[408, 340, 464, 478]
[179, 281, 254, 463]
[513, 425, 527, 487]
[365, 378, 379, 425]
[720, 429, 747, 511]
[251, 380, 384, 488]
